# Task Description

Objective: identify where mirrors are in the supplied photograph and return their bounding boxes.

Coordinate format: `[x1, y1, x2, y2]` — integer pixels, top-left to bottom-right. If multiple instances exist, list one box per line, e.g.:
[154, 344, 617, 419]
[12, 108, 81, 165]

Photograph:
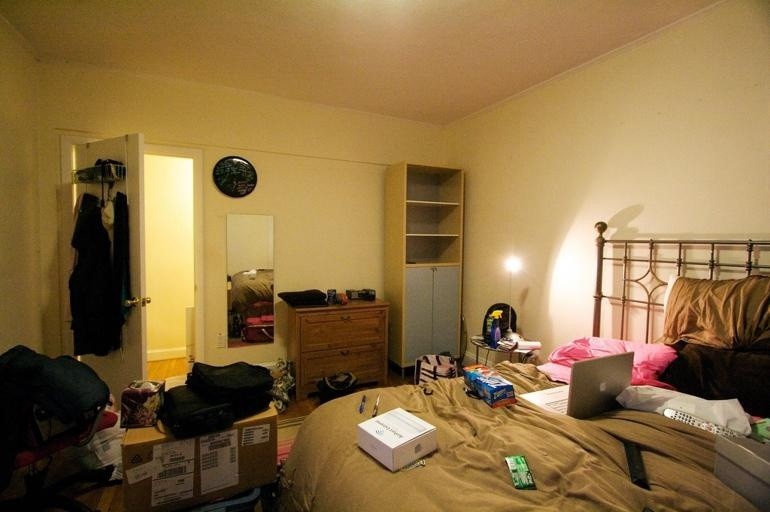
[226, 211, 275, 349]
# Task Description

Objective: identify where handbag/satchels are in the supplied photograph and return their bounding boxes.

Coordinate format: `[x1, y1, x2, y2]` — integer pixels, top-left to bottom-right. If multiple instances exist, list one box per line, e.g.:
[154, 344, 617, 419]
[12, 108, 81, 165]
[412, 351, 457, 388]
[119, 379, 165, 430]
[315, 370, 358, 407]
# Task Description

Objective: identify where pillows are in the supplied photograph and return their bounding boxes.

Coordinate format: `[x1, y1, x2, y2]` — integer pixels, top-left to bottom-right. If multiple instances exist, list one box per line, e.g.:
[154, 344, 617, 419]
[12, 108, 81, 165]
[657, 343, 770, 419]
[660, 275, 770, 357]
[548, 335, 678, 391]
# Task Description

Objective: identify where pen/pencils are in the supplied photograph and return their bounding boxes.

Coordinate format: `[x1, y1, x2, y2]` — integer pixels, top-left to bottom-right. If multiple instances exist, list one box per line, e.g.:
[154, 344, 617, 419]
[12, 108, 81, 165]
[372, 393, 380, 417]
[359, 395, 367, 414]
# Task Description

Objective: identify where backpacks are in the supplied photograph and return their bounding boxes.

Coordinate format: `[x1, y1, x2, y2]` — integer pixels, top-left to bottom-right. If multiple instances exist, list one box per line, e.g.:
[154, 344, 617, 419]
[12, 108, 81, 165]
[157, 358, 275, 438]
[33, 352, 111, 428]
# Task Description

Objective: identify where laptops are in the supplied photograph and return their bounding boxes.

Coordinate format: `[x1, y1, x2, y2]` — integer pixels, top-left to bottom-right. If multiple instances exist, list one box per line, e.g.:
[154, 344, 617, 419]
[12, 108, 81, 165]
[518, 351, 635, 417]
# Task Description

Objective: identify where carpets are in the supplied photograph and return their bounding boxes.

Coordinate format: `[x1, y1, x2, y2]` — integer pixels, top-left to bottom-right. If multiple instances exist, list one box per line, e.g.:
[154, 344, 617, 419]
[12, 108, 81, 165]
[277, 414, 309, 465]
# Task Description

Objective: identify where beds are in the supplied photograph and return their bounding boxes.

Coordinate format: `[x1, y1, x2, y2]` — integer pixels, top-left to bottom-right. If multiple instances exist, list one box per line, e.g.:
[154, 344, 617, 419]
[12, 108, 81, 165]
[229, 268, 275, 311]
[277, 221, 770, 512]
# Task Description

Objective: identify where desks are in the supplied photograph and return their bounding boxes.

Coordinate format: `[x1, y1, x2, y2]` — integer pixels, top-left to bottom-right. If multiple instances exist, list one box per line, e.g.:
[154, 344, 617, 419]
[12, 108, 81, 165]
[469, 333, 535, 367]
[0, 466, 120, 512]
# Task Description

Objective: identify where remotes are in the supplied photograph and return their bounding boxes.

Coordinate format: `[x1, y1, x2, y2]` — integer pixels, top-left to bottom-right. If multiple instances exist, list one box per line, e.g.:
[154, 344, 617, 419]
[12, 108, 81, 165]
[621, 439, 651, 490]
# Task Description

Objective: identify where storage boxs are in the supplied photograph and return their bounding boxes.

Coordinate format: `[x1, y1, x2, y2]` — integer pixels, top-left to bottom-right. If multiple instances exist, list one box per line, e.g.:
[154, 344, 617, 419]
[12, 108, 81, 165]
[712, 434, 770, 512]
[120, 400, 278, 512]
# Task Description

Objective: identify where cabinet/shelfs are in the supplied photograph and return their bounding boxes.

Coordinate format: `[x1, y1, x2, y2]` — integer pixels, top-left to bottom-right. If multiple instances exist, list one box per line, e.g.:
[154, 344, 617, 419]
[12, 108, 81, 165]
[287, 293, 391, 403]
[383, 159, 465, 380]
